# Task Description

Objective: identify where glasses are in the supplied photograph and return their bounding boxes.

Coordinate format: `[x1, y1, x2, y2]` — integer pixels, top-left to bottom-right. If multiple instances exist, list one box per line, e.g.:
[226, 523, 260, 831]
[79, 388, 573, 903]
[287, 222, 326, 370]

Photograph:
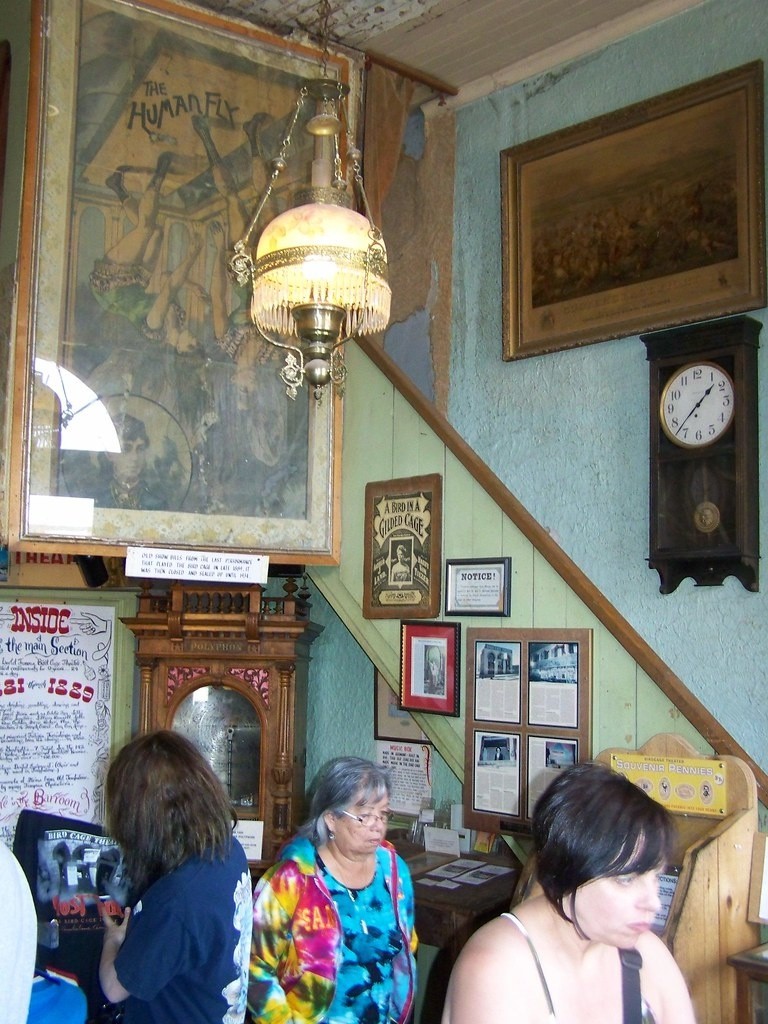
[334, 807, 395, 827]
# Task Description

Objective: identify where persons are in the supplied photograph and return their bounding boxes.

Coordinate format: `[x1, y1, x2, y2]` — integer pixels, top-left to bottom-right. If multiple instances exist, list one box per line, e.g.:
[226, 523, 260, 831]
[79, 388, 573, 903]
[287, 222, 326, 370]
[247, 757, 418, 1024]
[99, 730, 253, 1024]
[1, 840, 87, 1024]
[494, 747, 503, 760]
[391, 545, 410, 581]
[442, 761, 697, 1024]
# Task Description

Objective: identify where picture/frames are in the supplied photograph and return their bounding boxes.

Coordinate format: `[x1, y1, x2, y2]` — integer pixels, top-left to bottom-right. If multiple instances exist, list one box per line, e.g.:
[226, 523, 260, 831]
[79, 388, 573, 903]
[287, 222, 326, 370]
[398, 619, 461, 718]
[363, 473, 442, 618]
[445, 557, 511, 617]
[500, 58, 768, 363]
[7, 0, 352, 568]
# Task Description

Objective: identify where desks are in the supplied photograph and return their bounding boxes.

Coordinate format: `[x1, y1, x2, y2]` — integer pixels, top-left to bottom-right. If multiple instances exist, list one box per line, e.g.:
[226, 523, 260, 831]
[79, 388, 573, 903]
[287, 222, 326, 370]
[386, 828, 521, 1024]
[727, 939, 768, 1024]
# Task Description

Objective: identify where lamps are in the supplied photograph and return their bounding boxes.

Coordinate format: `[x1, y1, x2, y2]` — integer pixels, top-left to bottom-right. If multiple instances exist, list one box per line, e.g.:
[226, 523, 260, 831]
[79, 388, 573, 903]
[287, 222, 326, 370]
[229, 1, 392, 407]
[73, 555, 109, 588]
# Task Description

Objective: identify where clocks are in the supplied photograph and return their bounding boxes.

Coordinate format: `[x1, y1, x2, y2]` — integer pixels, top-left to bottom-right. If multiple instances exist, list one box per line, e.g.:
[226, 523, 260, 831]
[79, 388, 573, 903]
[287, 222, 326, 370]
[639, 314, 763, 592]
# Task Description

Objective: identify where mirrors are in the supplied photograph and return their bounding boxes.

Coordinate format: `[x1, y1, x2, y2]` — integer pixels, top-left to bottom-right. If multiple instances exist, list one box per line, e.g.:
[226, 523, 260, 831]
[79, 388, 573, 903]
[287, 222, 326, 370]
[163, 674, 268, 819]
